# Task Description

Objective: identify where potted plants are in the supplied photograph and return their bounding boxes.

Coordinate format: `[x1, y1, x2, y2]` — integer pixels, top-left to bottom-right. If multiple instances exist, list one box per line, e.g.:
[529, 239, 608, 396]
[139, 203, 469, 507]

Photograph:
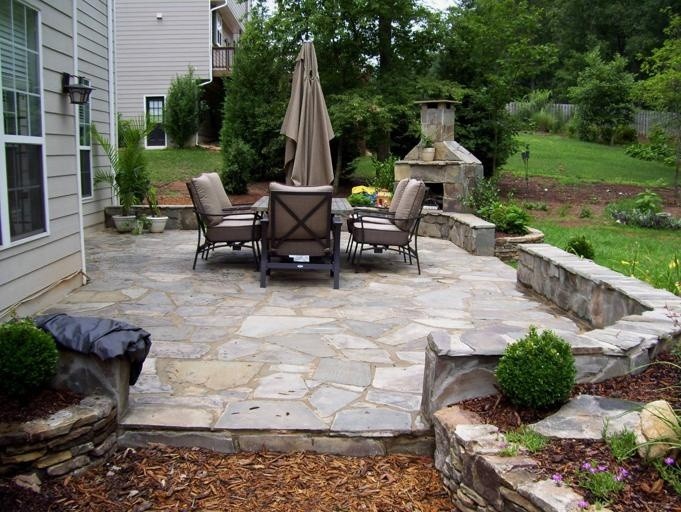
[420, 134, 435, 162]
[93, 112, 169, 233]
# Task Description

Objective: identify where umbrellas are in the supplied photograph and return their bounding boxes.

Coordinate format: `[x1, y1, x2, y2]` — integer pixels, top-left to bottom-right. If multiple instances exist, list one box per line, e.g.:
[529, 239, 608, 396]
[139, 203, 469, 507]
[278, 23, 336, 190]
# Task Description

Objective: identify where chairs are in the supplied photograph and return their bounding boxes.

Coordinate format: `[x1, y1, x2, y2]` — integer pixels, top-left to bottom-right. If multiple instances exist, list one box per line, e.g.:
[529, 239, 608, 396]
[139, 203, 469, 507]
[186, 172, 430, 289]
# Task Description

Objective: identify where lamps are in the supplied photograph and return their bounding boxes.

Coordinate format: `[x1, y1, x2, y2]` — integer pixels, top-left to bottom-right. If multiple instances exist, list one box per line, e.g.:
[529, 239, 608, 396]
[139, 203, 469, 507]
[62, 71, 97, 104]
